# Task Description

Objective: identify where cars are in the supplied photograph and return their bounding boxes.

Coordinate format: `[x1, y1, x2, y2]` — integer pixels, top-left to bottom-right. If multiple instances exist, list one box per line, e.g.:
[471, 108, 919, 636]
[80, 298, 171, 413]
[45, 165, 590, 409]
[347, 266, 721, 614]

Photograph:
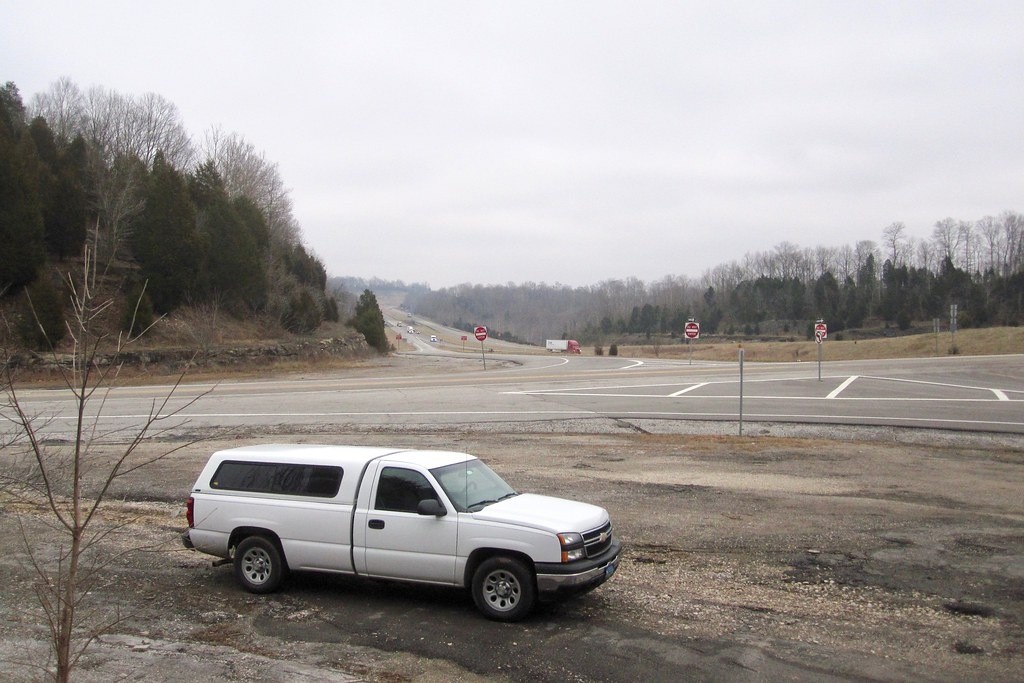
[417, 331, 421, 334]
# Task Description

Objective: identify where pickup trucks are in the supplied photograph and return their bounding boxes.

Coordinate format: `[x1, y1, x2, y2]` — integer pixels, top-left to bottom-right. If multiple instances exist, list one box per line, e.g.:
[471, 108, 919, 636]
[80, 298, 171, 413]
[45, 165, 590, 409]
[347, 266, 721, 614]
[181, 442, 622, 623]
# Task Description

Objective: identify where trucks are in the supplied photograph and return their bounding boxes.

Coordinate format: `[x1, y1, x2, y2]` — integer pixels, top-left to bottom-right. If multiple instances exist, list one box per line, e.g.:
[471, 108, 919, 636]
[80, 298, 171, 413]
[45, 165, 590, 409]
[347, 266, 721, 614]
[398, 321, 402, 327]
[546, 339, 581, 354]
[408, 313, 412, 318]
[407, 326, 414, 334]
[430, 335, 437, 342]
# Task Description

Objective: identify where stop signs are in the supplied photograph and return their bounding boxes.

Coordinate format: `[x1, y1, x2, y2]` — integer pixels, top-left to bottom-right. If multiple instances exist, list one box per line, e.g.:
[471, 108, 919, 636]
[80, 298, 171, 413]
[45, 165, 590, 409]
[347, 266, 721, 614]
[474, 326, 489, 341]
[685, 322, 700, 338]
[815, 323, 828, 339]
